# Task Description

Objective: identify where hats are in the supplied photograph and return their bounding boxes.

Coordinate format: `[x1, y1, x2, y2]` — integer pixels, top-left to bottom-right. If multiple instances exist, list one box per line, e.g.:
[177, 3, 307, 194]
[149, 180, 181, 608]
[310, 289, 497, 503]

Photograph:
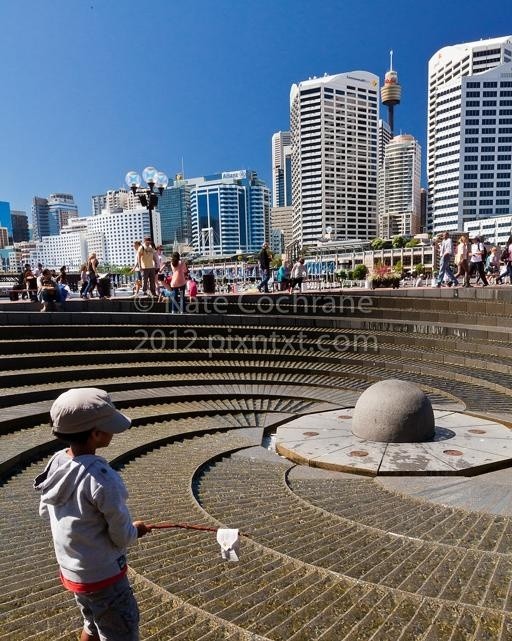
[50, 386, 132, 436]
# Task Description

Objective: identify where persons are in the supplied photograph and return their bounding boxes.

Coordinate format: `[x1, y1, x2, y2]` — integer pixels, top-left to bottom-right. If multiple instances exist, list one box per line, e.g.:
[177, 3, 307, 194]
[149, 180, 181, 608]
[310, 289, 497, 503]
[33, 386, 154, 641]
[13, 228, 511, 316]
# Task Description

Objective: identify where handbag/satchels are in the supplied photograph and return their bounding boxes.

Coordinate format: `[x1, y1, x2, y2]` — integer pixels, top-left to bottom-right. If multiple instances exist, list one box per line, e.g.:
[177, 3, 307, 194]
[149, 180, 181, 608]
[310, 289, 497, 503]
[477, 243, 488, 262]
[501, 247, 510, 261]
[454, 253, 464, 266]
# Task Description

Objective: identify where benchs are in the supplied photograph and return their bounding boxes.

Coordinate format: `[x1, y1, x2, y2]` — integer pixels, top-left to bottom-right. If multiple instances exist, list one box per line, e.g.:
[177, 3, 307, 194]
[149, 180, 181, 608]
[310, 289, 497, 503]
[7, 289, 38, 301]
[273, 280, 321, 292]
[447, 274, 500, 287]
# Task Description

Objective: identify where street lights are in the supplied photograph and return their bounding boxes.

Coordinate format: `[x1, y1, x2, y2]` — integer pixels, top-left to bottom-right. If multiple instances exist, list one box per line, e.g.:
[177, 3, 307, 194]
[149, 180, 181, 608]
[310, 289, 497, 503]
[125, 166, 169, 242]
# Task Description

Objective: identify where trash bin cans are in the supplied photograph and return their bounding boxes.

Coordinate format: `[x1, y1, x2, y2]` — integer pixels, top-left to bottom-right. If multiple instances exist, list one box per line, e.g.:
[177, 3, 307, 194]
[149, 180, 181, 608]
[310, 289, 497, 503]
[27, 276, 39, 301]
[97, 279, 111, 297]
[203, 274, 215, 293]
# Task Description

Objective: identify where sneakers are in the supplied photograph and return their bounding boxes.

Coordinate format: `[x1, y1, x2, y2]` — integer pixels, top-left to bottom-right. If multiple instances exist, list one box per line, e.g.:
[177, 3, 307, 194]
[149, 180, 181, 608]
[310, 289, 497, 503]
[257, 285, 273, 293]
